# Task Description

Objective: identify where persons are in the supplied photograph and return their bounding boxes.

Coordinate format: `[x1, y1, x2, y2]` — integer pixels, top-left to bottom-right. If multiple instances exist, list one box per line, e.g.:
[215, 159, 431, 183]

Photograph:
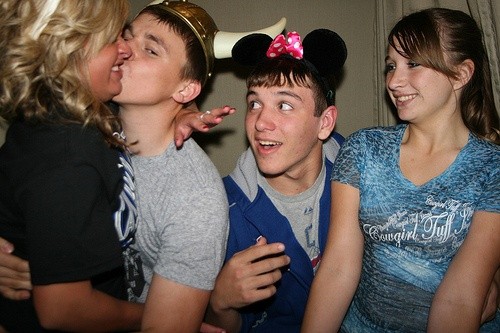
[203, 28, 500, 333]
[302, 8, 500, 333]
[0, 0, 287, 332]
[0, 0, 230, 333]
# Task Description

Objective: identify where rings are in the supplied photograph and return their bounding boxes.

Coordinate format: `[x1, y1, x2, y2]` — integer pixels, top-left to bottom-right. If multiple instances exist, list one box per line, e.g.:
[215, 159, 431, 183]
[201, 110, 210, 121]
